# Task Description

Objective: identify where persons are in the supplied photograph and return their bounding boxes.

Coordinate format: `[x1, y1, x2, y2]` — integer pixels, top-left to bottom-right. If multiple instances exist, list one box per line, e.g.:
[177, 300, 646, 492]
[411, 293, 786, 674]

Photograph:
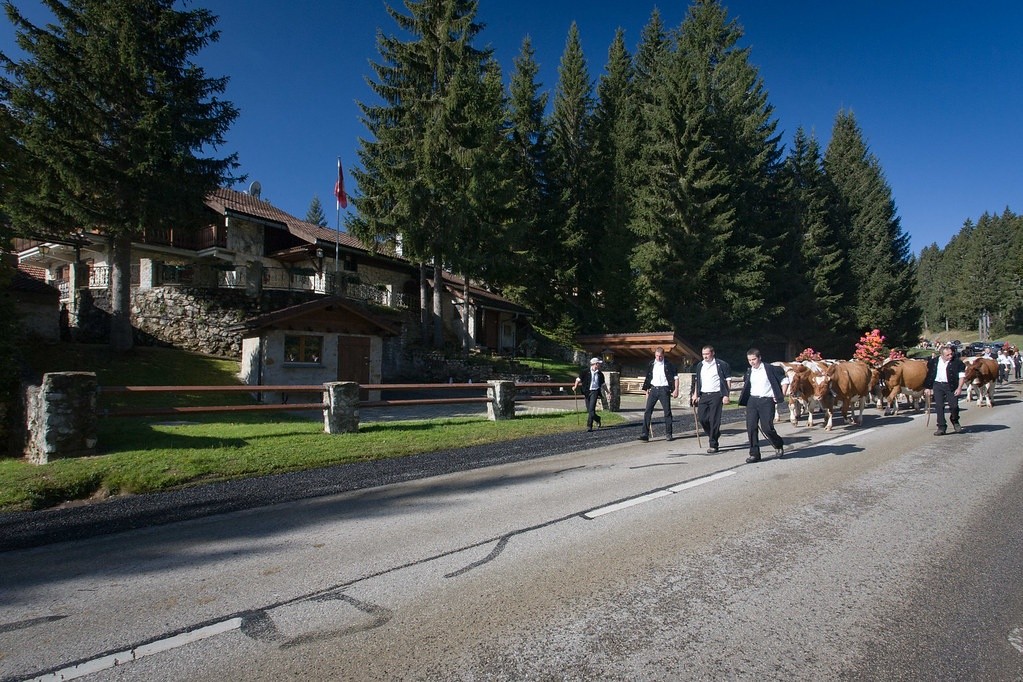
[636, 347, 679, 441]
[981, 347, 1023, 386]
[737, 348, 788, 463]
[691, 345, 731, 455]
[573, 357, 610, 433]
[925, 346, 966, 436]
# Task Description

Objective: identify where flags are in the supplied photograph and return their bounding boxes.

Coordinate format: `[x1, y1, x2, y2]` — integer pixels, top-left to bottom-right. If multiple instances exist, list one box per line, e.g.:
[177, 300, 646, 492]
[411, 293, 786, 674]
[333, 156, 351, 211]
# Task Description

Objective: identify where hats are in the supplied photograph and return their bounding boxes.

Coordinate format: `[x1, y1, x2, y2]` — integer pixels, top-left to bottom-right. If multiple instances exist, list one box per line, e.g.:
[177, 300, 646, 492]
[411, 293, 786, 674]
[590, 358, 602, 365]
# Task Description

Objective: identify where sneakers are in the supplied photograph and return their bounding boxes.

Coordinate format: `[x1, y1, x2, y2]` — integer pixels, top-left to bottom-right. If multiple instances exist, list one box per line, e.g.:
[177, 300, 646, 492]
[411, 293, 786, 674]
[746, 455, 761, 463]
[950, 418, 961, 433]
[776, 446, 783, 458]
[934, 424, 948, 436]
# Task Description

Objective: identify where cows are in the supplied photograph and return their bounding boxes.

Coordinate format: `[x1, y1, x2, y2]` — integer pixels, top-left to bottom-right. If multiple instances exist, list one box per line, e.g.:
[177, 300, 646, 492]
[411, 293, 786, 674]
[779, 356, 1000, 431]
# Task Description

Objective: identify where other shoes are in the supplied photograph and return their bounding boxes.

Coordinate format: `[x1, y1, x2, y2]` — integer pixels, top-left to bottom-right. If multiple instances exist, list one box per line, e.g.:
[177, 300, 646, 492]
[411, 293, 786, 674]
[637, 435, 649, 441]
[666, 434, 672, 441]
[707, 447, 718, 453]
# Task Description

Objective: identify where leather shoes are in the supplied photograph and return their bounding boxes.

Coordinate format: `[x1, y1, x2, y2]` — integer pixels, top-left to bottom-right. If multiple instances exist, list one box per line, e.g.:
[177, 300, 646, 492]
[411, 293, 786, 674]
[597, 416, 601, 428]
[587, 426, 592, 432]
